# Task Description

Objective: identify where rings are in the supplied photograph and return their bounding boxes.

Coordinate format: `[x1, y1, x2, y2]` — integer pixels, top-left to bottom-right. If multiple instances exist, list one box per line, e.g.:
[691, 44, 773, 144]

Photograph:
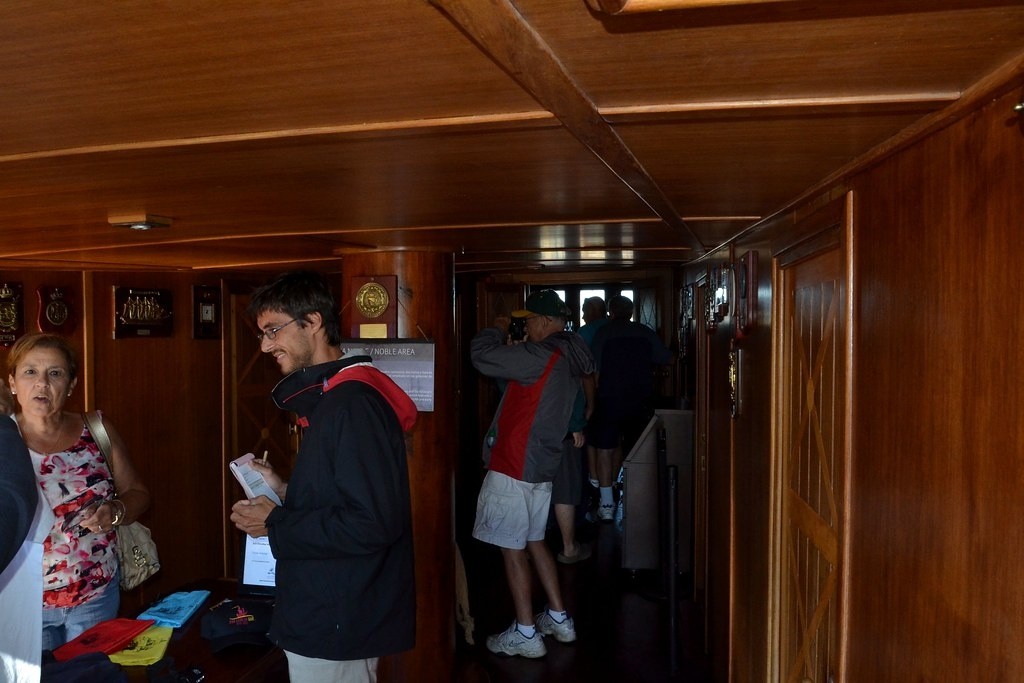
[99, 525, 103, 531]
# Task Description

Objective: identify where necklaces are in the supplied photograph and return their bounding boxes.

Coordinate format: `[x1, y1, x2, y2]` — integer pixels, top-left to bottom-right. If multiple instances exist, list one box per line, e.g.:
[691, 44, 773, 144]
[21, 411, 65, 458]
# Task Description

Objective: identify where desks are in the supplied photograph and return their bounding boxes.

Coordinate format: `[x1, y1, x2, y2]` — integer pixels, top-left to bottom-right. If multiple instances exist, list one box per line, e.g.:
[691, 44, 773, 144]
[120, 581, 285, 683]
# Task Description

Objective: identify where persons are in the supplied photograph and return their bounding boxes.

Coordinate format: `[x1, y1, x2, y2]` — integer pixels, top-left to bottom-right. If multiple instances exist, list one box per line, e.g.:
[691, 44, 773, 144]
[468, 288, 668, 659]
[0, 334, 152, 652]
[231, 271, 421, 683]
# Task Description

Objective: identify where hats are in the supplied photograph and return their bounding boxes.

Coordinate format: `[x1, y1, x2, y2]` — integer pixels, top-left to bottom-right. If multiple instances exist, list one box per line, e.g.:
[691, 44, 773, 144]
[512, 290, 572, 318]
[201, 599, 272, 653]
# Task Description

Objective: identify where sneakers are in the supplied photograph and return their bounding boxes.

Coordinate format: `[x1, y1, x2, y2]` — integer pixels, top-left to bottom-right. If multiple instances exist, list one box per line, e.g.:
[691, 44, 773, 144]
[598, 500, 616, 520]
[487, 618, 547, 657]
[534, 609, 577, 642]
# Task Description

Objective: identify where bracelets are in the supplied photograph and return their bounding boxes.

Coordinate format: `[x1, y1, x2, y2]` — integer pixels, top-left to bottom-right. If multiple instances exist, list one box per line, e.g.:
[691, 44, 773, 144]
[112, 498, 127, 524]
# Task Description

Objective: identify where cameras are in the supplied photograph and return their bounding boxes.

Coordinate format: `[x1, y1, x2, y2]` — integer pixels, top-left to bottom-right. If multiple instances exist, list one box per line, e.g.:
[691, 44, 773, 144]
[508, 318, 527, 341]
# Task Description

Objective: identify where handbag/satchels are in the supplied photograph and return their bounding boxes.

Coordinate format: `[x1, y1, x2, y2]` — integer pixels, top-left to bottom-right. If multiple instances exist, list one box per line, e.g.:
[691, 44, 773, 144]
[76, 411, 162, 593]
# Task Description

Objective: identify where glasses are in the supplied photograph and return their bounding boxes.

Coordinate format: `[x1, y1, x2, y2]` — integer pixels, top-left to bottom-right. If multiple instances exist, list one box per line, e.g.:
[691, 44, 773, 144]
[521, 314, 551, 324]
[256, 317, 301, 340]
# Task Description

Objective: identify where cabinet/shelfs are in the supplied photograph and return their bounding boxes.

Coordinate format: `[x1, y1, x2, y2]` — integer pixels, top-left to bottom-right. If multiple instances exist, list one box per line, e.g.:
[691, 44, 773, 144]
[620, 406, 696, 577]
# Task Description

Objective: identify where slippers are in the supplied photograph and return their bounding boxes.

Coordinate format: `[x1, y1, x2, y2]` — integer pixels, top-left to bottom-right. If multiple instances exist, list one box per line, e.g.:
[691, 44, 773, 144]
[557, 541, 592, 563]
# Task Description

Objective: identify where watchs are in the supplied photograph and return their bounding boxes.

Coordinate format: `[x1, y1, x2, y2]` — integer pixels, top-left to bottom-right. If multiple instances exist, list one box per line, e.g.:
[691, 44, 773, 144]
[107, 499, 121, 526]
